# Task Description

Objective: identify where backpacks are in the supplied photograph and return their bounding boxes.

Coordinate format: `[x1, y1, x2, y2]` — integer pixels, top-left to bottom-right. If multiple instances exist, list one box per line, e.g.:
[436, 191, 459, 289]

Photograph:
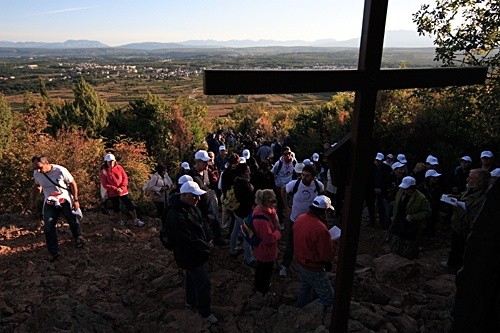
[223, 186, 240, 211]
[239, 214, 269, 247]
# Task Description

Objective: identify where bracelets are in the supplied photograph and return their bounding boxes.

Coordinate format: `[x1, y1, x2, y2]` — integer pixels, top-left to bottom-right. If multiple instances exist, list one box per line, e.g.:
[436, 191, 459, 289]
[31, 201, 37, 203]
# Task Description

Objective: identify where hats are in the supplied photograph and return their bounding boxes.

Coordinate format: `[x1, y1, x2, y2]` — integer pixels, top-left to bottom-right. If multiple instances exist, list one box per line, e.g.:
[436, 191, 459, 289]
[397, 154, 407, 163]
[313, 153, 319, 161]
[195, 150, 211, 161]
[243, 149, 250, 159]
[490, 168, 500, 176]
[391, 162, 405, 170]
[303, 159, 310, 164]
[219, 146, 226, 150]
[104, 154, 116, 161]
[480, 151, 493, 158]
[179, 180, 207, 195]
[424, 169, 442, 178]
[182, 162, 190, 169]
[178, 175, 194, 184]
[376, 153, 384, 160]
[295, 163, 305, 173]
[426, 155, 438, 164]
[398, 176, 416, 188]
[460, 156, 472, 162]
[239, 157, 246, 163]
[312, 195, 335, 211]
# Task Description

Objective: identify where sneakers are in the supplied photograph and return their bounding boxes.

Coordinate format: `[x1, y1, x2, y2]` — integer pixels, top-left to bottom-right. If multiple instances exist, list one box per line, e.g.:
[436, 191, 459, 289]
[207, 314, 218, 323]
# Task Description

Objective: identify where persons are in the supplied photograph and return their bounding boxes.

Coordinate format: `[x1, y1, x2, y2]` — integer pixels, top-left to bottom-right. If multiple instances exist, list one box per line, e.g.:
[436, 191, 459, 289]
[100, 152, 145, 226]
[31, 155, 85, 261]
[146, 126, 500, 333]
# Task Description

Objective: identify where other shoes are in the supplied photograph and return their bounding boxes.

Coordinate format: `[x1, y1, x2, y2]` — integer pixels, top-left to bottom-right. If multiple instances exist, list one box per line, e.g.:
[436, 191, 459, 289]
[76, 238, 85, 248]
[134, 220, 145, 227]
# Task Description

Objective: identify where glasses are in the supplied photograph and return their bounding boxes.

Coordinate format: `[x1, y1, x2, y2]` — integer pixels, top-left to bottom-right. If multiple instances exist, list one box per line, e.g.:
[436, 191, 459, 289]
[284, 151, 292, 156]
